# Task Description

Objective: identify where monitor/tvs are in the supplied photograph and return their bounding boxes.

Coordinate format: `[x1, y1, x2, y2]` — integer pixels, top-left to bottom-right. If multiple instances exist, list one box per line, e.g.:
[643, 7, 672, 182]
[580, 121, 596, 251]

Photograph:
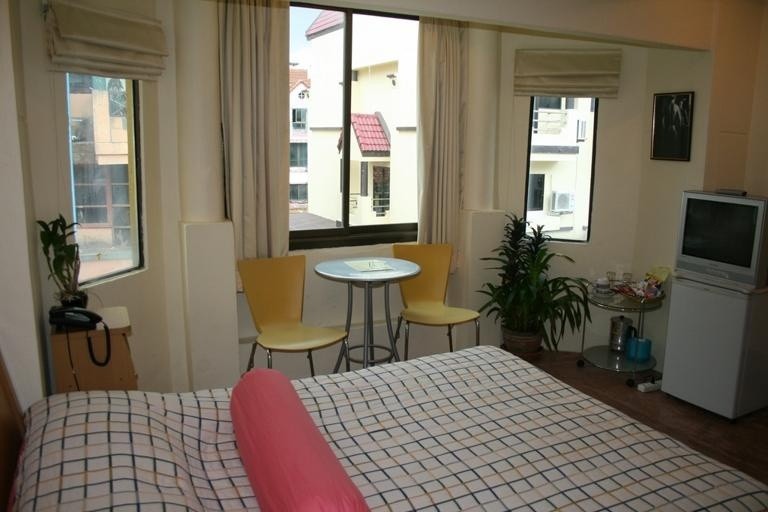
[675, 190, 764, 294]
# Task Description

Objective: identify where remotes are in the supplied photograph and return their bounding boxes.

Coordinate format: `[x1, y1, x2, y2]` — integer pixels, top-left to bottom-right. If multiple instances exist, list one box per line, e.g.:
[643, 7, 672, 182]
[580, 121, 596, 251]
[717, 189, 748, 196]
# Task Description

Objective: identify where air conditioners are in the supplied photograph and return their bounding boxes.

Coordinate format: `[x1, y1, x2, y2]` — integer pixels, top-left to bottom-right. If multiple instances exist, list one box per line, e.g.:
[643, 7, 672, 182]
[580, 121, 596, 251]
[577, 119, 588, 143]
[551, 190, 576, 216]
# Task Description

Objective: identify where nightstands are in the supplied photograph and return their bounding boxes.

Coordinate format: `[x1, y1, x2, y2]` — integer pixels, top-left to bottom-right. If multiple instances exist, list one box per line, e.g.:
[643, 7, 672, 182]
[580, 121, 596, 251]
[49, 307, 138, 394]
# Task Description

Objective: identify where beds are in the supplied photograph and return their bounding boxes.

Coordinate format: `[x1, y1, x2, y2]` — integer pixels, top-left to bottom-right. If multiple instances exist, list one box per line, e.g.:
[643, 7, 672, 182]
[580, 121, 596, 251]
[0, 344, 767, 512]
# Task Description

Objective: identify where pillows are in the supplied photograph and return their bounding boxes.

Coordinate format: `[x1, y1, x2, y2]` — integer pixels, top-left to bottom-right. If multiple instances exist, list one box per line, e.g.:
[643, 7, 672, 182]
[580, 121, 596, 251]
[230, 368, 370, 511]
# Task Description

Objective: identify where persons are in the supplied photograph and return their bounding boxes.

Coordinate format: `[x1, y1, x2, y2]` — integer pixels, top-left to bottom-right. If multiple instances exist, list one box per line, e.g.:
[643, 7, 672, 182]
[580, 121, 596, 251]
[680, 97, 690, 126]
[661, 94, 682, 143]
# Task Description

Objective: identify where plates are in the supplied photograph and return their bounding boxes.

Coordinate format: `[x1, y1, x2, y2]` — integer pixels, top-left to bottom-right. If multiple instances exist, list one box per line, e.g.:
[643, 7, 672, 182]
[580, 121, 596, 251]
[595, 291, 614, 298]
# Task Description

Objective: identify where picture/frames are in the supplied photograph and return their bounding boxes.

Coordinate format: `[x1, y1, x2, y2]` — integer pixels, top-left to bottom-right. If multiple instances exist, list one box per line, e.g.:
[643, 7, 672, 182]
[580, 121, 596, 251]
[650, 91, 695, 162]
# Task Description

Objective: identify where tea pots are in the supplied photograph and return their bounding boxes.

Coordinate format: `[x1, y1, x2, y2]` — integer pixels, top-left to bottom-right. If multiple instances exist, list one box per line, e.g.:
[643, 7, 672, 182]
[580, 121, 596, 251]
[597, 277, 609, 294]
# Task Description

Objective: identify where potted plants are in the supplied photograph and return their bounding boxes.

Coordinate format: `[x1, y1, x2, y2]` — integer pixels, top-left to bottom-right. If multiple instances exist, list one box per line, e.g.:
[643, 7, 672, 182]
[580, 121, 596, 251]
[36, 214, 88, 308]
[477, 212, 593, 362]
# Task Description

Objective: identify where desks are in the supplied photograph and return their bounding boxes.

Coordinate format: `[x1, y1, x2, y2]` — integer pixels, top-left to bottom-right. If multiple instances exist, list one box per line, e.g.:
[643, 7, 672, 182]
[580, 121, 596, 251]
[577, 281, 666, 387]
[314, 258, 421, 374]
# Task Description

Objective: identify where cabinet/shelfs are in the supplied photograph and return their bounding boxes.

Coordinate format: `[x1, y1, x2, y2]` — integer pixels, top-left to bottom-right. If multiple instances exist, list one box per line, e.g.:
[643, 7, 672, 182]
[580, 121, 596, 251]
[661, 278, 768, 420]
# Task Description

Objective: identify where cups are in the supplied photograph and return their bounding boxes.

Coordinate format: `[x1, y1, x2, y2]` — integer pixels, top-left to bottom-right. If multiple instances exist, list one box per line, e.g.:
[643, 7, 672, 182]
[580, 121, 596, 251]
[606, 271, 616, 289]
[623, 273, 632, 285]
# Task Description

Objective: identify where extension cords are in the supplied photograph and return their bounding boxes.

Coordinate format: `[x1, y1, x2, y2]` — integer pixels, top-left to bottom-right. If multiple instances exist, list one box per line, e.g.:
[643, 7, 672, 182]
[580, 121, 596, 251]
[638, 379, 662, 392]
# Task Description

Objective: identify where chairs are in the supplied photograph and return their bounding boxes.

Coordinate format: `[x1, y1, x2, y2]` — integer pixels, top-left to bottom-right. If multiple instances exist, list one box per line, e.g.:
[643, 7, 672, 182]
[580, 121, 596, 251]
[239, 255, 350, 377]
[388, 243, 480, 363]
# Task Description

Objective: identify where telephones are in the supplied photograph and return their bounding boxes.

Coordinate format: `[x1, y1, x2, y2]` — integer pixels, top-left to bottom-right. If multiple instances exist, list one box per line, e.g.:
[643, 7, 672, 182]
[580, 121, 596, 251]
[48, 306, 109, 366]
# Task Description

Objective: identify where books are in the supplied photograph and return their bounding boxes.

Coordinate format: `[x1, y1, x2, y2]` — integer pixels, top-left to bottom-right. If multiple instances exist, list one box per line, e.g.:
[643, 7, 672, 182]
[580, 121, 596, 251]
[344, 259, 394, 271]
